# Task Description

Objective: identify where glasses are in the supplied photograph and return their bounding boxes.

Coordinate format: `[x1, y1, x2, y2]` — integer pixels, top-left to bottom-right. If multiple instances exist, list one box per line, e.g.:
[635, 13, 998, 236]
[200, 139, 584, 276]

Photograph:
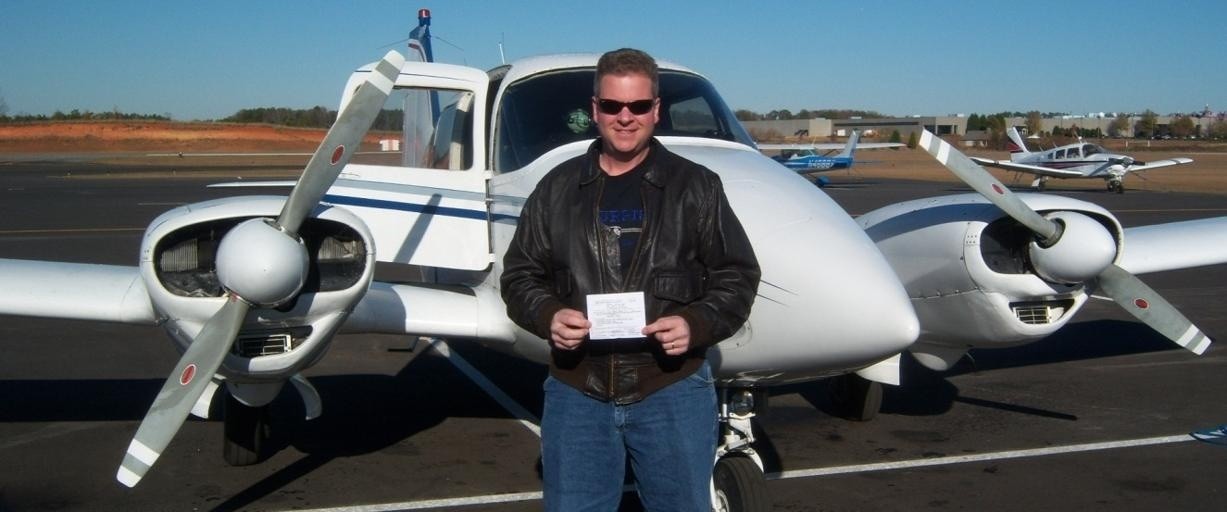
[596, 94, 660, 117]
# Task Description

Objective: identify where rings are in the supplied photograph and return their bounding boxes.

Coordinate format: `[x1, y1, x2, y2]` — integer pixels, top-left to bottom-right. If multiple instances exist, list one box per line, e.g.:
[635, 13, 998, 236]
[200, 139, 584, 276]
[670, 341, 676, 351]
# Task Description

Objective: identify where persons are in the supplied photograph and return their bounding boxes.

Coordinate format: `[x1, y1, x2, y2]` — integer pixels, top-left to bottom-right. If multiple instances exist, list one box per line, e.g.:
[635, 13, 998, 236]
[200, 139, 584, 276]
[496, 45, 762, 512]
